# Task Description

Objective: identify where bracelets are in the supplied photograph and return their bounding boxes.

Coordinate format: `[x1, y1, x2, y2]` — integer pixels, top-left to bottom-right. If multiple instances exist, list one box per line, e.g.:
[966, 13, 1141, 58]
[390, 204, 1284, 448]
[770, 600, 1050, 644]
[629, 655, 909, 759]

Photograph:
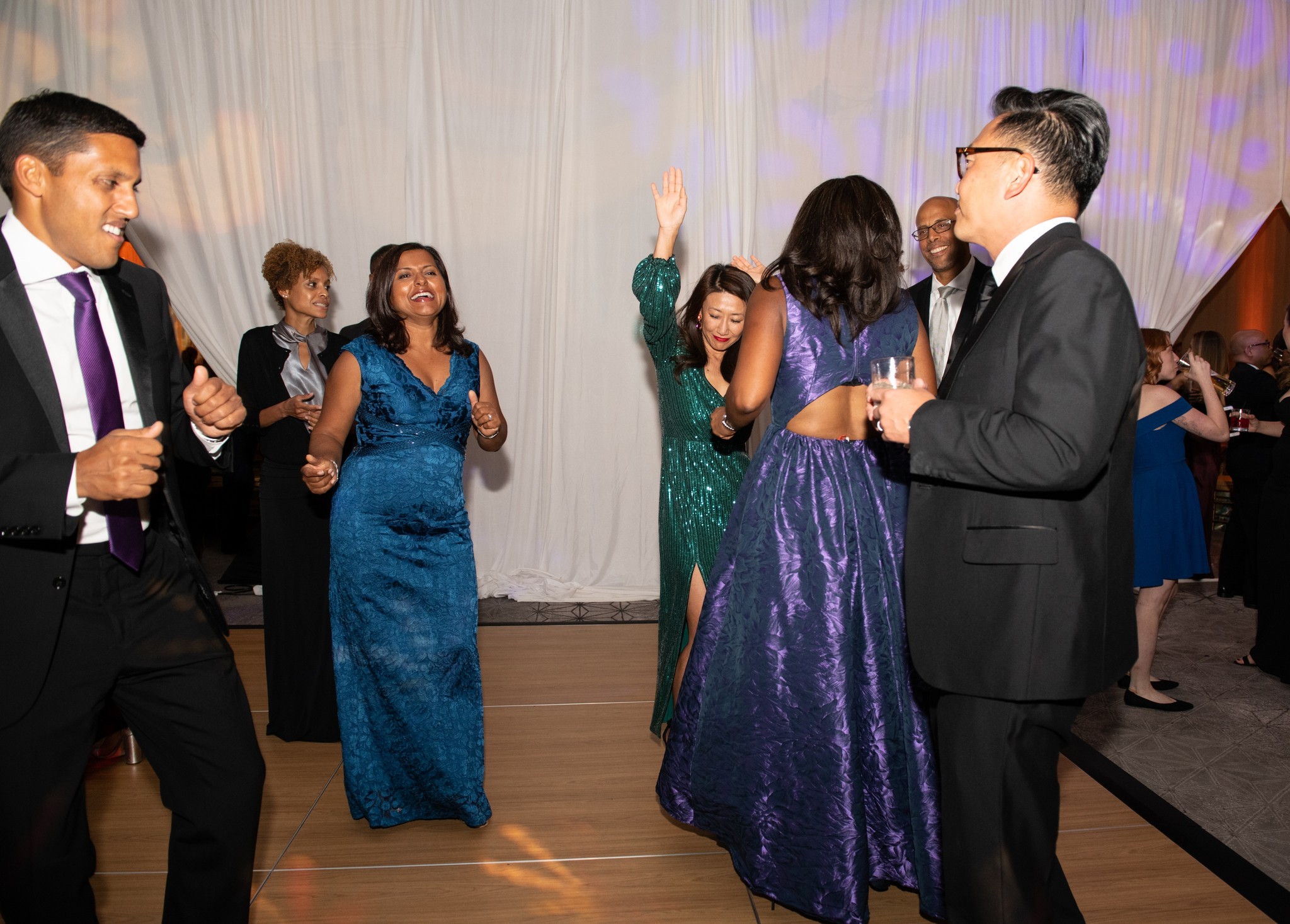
[191, 421, 227, 443]
[330, 460, 339, 482]
[477, 428, 499, 439]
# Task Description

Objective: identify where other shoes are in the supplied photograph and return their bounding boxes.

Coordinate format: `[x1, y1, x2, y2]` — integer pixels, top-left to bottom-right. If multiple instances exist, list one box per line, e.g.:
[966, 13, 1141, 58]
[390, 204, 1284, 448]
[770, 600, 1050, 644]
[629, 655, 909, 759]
[1117, 674, 1179, 691]
[1123, 689, 1194, 711]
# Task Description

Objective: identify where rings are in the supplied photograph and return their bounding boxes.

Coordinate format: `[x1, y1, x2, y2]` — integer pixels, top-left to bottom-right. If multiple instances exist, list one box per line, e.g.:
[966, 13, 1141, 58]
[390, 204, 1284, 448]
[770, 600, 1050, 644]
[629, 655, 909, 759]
[751, 266, 755, 269]
[488, 413, 492, 420]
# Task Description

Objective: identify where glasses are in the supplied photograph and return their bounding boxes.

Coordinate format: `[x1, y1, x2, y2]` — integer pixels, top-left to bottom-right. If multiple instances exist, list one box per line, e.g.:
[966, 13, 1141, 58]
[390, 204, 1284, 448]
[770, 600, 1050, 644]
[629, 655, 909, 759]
[1244, 342, 1270, 352]
[912, 219, 956, 241]
[956, 147, 1039, 179]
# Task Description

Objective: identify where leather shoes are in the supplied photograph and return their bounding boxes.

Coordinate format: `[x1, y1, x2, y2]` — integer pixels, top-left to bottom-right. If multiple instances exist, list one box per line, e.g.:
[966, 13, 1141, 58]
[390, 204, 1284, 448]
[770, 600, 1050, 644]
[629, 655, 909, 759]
[1217, 584, 1234, 597]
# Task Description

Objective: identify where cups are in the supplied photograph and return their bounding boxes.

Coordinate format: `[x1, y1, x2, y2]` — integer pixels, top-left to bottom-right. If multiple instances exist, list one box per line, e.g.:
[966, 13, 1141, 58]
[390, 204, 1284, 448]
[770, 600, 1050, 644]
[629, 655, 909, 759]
[870, 356, 915, 393]
[1176, 352, 1236, 397]
[1230, 408, 1250, 432]
[1272, 348, 1284, 361]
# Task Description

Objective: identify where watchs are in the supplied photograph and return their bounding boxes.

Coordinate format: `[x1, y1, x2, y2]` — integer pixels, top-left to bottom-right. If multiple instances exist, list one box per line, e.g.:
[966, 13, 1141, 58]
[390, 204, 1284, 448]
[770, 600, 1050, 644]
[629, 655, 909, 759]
[722, 413, 736, 431]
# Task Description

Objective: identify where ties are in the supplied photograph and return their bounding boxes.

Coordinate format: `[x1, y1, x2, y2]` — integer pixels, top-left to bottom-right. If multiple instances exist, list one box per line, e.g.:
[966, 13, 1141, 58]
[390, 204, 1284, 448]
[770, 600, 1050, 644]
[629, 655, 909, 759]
[972, 269, 997, 329]
[56, 271, 146, 570]
[929, 286, 956, 389]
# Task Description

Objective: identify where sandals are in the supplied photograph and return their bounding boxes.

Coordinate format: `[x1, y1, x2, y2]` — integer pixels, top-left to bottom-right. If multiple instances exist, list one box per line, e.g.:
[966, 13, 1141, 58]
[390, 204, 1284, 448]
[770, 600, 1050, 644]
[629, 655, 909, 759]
[1233, 655, 1258, 667]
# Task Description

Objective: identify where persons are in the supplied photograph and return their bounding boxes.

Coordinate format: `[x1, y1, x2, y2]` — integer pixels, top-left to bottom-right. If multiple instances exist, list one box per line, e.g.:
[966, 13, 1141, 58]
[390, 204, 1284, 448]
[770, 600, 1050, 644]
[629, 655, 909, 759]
[1118, 305, 1290, 711]
[0, 94, 267, 924]
[632, 87, 1147, 924]
[236, 240, 508, 830]
